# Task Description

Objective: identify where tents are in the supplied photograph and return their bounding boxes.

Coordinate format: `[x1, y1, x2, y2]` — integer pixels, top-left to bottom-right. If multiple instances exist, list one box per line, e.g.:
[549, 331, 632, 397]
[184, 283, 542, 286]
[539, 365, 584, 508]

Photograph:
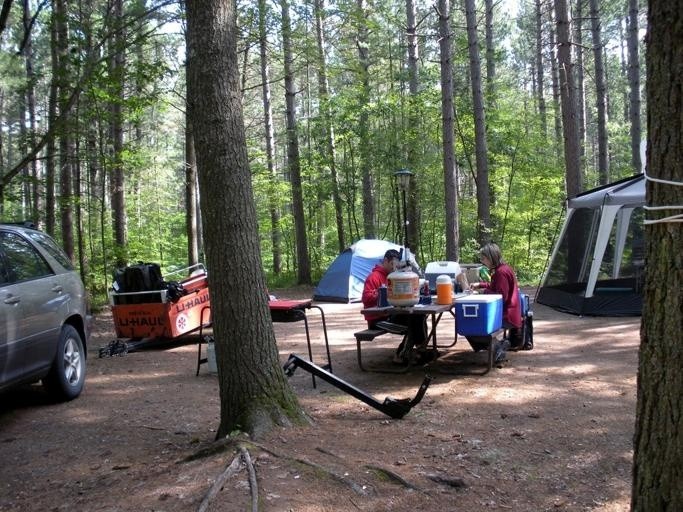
[535, 171, 652, 317]
[314, 237, 415, 303]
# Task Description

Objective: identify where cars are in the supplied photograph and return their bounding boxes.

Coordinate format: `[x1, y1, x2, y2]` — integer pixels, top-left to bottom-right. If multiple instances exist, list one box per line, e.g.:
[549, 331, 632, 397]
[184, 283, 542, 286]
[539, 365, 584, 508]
[0, 222, 92, 398]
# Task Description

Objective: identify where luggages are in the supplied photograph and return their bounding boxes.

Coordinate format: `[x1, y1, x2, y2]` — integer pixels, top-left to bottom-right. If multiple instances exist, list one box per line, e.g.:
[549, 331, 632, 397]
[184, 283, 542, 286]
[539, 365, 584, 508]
[520, 294, 526, 317]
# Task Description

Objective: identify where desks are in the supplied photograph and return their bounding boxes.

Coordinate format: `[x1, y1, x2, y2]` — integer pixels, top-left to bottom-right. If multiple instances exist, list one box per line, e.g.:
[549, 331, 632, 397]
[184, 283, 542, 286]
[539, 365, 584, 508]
[359, 290, 471, 375]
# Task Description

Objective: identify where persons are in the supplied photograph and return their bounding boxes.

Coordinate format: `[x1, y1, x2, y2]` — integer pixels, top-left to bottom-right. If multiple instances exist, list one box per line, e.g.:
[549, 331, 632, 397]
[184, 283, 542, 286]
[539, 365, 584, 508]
[362, 249, 427, 364]
[467, 243, 521, 362]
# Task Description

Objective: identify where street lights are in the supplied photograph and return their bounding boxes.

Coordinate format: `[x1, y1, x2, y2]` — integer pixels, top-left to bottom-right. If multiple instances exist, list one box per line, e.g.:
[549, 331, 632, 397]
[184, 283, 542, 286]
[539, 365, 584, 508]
[393, 169, 414, 247]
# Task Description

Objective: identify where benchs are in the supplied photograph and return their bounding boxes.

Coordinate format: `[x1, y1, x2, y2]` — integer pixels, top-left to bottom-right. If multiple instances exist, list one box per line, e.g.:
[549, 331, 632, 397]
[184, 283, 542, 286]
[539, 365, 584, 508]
[353, 327, 523, 374]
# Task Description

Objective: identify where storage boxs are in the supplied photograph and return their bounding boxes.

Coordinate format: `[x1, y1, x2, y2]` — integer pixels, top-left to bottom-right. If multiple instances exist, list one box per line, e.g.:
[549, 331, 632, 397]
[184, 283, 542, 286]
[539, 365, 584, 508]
[453, 293, 503, 337]
